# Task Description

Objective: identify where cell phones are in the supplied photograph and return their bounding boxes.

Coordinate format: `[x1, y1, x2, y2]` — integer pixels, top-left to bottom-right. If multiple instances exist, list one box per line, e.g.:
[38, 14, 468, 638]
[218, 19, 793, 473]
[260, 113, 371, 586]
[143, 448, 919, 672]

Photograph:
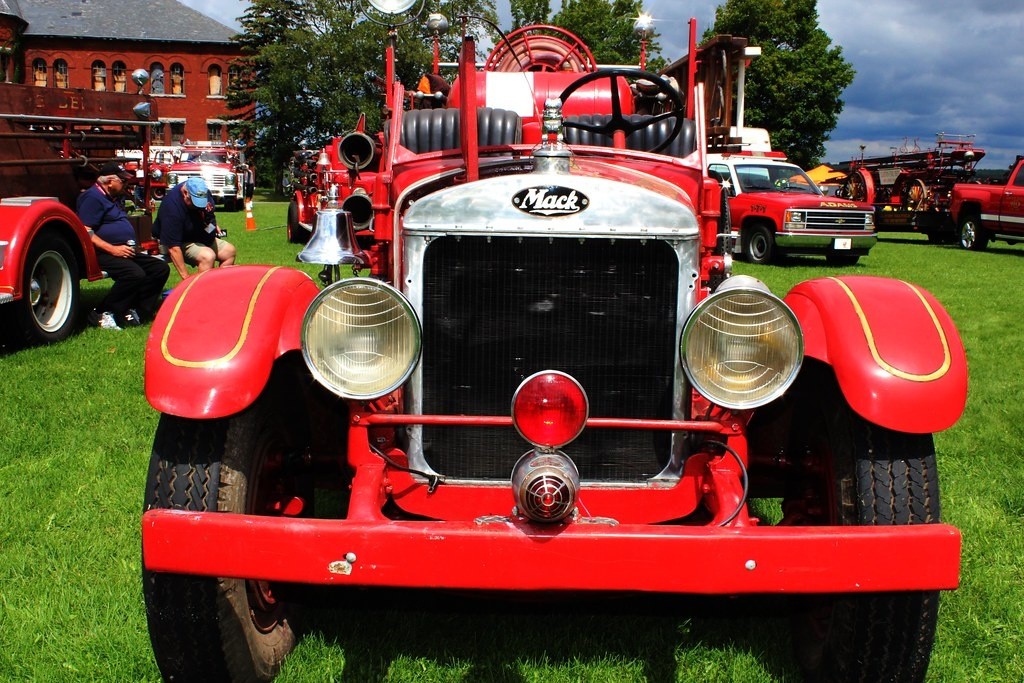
[214, 229, 228, 236]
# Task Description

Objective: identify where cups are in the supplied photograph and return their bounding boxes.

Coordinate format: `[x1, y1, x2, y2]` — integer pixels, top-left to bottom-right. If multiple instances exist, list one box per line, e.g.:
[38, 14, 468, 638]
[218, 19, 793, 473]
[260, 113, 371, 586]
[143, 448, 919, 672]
[127, 240, 136, 250]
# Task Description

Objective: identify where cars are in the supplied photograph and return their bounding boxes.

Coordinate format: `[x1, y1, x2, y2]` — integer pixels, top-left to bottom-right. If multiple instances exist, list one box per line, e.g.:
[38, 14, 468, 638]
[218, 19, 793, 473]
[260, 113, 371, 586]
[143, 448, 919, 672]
[0, 101, 154, 344]
[140, 18, 969, 681]
[949, 153, 1024, 250]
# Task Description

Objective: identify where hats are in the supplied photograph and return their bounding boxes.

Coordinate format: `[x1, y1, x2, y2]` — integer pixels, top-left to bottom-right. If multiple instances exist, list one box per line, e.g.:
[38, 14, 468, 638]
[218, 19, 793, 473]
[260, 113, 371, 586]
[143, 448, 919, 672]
[187, 178, 208, 208]
[99, 162, 134, 180]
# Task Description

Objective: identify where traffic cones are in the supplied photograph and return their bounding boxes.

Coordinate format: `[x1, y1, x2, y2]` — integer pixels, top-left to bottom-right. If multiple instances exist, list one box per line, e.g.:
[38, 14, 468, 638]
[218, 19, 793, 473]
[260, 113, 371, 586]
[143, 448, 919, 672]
[243, 197, 256, 233]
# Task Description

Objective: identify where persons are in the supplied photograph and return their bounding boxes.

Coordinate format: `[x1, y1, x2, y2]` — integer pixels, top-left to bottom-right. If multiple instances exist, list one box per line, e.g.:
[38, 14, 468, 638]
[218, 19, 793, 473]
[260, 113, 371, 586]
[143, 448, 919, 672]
[225, 132, 248, 171]
[152, 178, 236, 282]
[75, 164, 170, 332]
[282, 173, 290, 197]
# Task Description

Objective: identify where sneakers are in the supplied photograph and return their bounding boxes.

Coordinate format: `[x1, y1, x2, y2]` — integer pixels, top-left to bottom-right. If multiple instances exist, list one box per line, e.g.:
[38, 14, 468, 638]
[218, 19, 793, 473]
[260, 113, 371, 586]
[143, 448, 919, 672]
[88, 308, 123, 332]
[121, 309, 142, 326]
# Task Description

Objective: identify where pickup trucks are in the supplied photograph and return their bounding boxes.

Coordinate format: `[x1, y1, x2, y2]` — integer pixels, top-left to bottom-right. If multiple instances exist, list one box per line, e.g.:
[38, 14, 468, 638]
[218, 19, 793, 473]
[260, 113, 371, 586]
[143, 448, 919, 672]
[152, 141, 254, 211]
